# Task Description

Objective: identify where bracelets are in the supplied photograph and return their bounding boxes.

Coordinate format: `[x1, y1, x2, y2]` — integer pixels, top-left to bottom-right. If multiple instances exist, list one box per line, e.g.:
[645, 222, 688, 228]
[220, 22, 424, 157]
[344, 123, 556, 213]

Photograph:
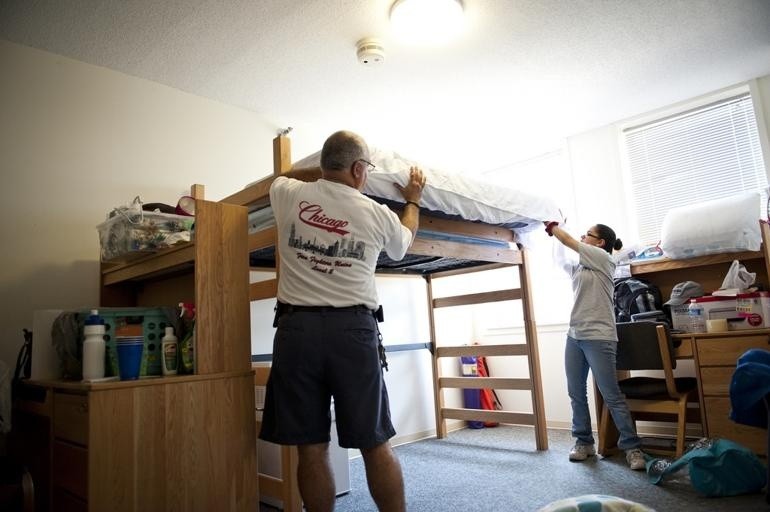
[545, 222, 558, 236]
[407, 201, 420, 208]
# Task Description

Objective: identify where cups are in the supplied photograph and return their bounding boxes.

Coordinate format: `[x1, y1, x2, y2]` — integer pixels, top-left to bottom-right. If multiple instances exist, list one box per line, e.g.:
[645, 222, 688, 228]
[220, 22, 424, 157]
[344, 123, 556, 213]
[115, 335, 143, 381]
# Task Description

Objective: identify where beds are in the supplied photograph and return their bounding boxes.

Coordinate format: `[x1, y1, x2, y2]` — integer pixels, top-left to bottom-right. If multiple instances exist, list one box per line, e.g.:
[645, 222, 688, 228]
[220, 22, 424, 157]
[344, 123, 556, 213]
[190, 136, 563, 512]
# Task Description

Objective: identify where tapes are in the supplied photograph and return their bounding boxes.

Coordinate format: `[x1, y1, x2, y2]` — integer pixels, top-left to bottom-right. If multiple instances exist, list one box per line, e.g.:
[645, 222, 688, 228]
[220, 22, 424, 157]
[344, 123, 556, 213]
[707, 319, 729, 334]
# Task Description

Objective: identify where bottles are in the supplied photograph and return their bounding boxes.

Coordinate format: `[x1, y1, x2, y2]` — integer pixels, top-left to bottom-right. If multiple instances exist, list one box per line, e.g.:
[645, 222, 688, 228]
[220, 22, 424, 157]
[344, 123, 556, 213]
[81, 309, 107, 380]
[688, 299, 705, 333]
[161, 327, 181, 376]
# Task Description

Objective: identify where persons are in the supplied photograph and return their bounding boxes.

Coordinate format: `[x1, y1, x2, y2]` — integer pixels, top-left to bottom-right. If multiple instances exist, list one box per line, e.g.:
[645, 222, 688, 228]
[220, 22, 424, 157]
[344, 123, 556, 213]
[258, 130, 427, 512]
[543, 221, 645, 470]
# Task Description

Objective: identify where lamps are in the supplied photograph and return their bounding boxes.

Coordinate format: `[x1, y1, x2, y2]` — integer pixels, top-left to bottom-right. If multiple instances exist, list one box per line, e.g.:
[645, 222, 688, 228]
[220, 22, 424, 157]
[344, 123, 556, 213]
[388, 0, 463, 37]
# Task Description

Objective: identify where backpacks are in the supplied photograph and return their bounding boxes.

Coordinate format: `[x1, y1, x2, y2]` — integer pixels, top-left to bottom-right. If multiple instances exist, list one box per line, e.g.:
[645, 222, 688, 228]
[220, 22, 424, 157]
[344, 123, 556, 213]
[612, 277, 662, 323]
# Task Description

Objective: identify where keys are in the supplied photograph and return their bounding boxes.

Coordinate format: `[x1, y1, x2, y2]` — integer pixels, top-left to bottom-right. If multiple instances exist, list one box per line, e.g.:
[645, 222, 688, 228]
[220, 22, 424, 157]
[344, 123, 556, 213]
[378, 344, 388, 371]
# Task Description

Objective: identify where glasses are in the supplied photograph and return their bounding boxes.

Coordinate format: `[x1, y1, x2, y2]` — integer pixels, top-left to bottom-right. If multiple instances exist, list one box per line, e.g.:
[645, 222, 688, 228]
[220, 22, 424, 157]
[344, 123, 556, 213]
[359, 158, 375, 172]
[586, 230, 600, 240]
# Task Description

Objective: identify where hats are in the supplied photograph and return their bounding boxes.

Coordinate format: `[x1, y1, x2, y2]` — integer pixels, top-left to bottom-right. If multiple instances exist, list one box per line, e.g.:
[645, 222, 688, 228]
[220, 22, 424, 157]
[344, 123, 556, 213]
[662, 281, 705, 307]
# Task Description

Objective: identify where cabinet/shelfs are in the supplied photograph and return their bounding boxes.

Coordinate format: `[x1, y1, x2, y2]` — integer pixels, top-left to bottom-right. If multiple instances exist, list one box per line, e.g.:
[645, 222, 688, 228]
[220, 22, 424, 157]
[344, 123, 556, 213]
[250, 360, 352, 510]
[11, 200, 260, 512]
[591, 219, 770, 463]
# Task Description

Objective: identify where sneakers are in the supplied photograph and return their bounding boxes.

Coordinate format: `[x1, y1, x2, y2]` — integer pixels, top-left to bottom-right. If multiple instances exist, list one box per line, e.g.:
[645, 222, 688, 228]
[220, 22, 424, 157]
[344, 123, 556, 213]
[625, 448, 646, 469]
[569, 443, 596, 460]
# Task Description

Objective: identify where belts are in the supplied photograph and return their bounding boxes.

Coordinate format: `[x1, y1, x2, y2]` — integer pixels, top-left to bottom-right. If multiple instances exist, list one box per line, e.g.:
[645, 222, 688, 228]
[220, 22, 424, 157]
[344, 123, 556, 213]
[277, 303, 375, 315]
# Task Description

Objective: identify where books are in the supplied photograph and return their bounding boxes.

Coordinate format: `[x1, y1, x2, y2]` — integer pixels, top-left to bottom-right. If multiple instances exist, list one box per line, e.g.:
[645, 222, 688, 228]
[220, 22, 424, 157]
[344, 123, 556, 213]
[712, 287, 738, 296]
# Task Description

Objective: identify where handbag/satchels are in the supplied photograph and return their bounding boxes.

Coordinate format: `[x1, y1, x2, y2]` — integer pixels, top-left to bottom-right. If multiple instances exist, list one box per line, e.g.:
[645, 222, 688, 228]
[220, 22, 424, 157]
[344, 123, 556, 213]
[688, 439, 768, 498]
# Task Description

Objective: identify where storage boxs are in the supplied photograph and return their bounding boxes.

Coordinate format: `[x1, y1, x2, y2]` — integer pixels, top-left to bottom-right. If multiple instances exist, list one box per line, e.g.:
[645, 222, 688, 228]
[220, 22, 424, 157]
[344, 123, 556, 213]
[95, 209, 196, 266]
[667, 291, 769, 334]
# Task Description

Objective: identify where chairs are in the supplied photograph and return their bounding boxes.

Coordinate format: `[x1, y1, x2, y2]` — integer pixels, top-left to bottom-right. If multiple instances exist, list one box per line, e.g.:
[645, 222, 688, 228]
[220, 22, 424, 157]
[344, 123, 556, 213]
[598, 319, 698, 463]
